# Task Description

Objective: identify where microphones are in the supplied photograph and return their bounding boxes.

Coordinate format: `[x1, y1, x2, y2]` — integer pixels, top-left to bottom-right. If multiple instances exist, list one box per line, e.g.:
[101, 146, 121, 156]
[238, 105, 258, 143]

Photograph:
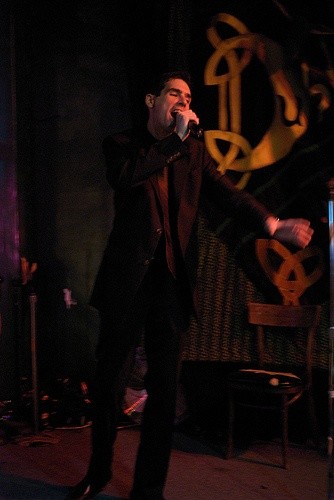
[174, 112, 203, 137]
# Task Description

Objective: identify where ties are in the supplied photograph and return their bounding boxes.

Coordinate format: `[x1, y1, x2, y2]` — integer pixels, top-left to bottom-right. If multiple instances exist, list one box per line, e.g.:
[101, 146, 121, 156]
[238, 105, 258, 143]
[151, 166, 177, 281]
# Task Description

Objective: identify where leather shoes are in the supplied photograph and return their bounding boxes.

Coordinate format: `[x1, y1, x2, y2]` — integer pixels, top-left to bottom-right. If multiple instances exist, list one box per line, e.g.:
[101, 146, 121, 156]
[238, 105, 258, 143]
[66, 471, 114, 500]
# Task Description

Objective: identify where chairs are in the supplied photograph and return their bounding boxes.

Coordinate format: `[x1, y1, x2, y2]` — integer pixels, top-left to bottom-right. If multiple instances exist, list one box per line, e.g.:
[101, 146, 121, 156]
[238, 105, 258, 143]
[226, 303, 320, 469]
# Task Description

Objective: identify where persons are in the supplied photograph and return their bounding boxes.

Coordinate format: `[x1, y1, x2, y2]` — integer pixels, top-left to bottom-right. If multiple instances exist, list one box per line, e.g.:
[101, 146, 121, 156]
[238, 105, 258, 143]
[67, 70, 314, 499]
